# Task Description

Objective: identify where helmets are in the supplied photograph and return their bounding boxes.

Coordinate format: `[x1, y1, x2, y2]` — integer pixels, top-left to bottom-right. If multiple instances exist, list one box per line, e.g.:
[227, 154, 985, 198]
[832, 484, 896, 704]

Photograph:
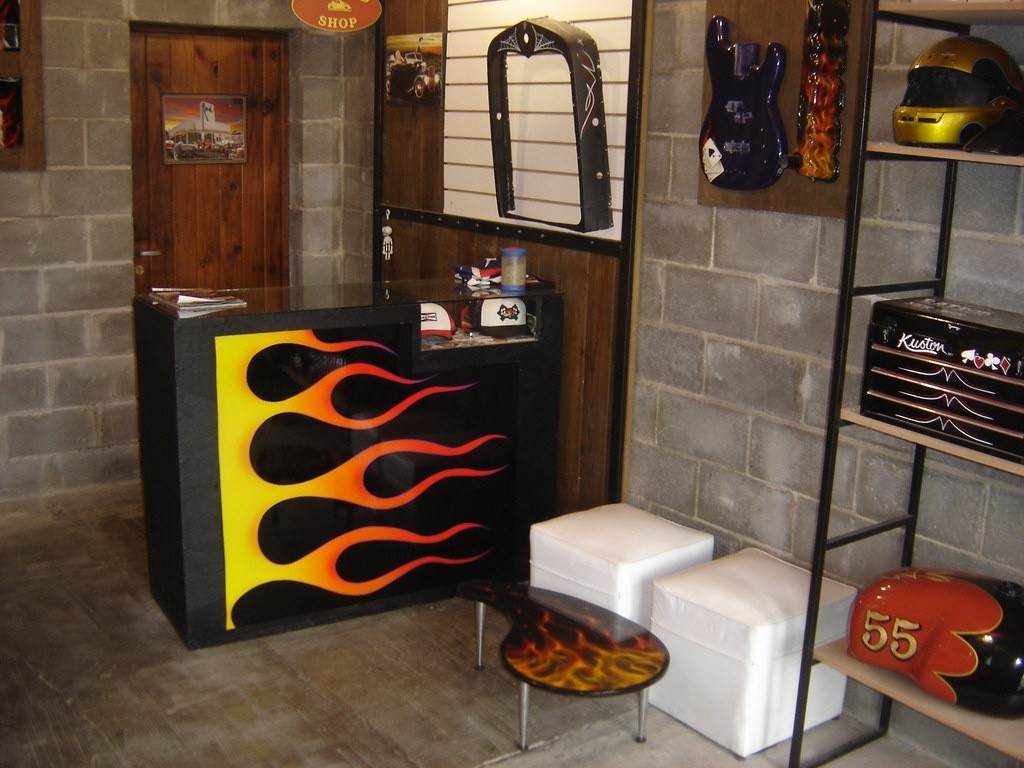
[892, 35, 1024, 149]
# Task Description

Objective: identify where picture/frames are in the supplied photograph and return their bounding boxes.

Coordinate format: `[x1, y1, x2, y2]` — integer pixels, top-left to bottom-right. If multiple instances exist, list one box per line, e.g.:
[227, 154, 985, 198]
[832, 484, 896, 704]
[0, 0, 47, 172]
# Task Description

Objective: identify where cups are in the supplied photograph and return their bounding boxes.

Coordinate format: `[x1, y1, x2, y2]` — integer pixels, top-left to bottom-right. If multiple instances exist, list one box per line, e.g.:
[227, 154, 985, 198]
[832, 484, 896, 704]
[501, 248, 527, 292]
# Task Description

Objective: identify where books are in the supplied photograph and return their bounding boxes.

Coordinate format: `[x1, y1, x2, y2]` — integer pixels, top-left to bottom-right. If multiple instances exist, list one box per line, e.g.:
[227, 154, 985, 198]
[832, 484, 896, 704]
[150, 287, 247, 311]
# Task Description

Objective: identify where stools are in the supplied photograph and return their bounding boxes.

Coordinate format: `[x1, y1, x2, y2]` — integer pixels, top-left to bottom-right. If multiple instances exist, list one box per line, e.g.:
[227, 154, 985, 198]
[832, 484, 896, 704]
[648, 546, 858, 757]
[529, 503, 714, 632]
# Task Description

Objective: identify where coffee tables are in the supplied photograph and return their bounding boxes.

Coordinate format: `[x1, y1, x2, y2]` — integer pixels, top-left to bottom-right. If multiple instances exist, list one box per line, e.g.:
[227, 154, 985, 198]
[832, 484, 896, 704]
[457, 579, 670, 751]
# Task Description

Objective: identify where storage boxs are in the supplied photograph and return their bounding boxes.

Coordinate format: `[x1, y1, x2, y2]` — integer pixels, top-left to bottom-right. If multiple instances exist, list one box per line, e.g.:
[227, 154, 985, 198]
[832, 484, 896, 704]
[859, 296, 1024, 465]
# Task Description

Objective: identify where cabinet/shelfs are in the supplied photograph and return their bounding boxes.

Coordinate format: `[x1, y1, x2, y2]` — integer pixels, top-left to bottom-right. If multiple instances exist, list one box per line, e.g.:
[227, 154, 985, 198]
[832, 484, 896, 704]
[789, 0, 1024, 768]
[132, 277, 567, 650]
